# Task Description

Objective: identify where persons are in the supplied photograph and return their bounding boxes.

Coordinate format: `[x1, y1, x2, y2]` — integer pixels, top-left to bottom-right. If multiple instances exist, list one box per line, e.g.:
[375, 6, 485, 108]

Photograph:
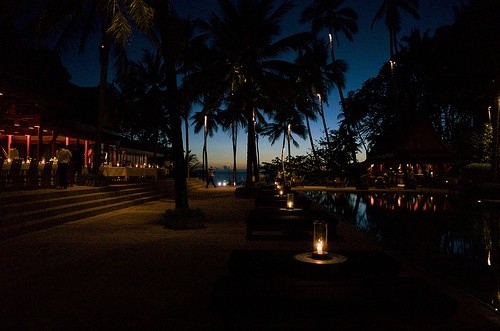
[9, 142, 19, 160]
[206, 166, 217, 188]
[71, 138, 84, 174]
[56, 141, 72, 188]
[370, 167, 416, 188]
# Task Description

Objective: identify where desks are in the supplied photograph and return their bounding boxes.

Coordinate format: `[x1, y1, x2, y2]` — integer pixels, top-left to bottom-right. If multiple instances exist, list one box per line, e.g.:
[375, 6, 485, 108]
[226, 161, 438, 306]
[0, 155, 170, 189]
